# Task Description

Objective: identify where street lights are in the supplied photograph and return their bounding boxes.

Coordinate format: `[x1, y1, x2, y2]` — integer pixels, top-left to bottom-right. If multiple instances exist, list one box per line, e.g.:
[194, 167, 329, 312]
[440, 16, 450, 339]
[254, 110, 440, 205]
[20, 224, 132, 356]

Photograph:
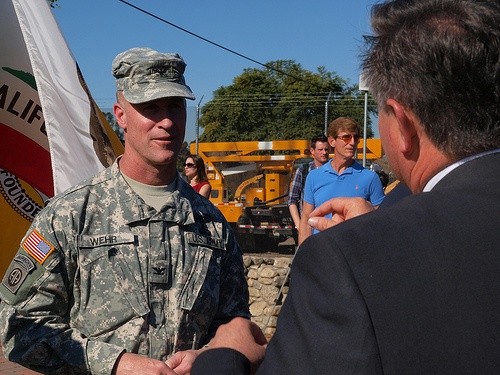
[324, 84, 343, 138]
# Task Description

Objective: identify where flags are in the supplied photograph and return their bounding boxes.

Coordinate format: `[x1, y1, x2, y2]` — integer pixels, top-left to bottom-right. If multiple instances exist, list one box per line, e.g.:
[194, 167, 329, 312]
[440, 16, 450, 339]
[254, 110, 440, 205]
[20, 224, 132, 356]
[0, 2, 123, 282]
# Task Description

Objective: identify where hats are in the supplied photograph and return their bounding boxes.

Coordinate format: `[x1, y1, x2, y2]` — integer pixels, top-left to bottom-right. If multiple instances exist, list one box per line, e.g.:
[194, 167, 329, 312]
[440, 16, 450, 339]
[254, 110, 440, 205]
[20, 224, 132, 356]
[111, 48, 195, 104]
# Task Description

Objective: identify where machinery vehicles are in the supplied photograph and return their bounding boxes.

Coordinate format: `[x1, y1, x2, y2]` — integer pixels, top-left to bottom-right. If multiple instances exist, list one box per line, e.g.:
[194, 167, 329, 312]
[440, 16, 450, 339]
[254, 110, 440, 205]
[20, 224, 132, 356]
[188, 135, 391, 252]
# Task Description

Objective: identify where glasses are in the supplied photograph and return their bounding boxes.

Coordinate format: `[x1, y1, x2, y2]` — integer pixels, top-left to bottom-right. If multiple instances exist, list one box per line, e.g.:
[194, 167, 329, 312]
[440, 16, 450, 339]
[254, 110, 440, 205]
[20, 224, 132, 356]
[337, 134, 360, 144]
[183, 163, 196, 168]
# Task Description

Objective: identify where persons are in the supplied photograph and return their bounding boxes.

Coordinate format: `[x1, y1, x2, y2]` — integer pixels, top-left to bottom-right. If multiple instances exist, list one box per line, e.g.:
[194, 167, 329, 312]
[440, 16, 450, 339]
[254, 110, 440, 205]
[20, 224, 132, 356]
[0, 48, 251, 375]
[288, 135, 329, 229]
[190, 0, 500, 375]
[298, 118, 386, 246]
[185, 155, 211, 200]
